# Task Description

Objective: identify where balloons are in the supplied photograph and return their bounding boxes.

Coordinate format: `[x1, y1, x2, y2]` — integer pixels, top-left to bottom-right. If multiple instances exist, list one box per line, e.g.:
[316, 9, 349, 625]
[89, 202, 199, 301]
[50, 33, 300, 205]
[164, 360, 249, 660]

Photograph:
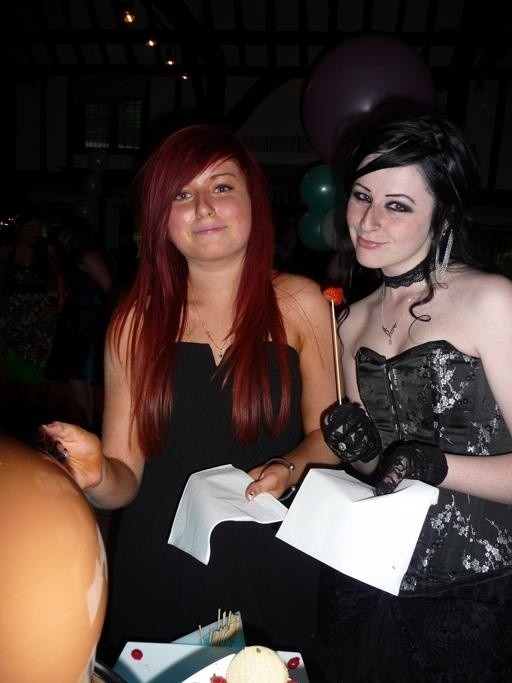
[78, 199, 96, 224]
[86, 174, 105, 197]
[303, 35, 438, 165]
[89, 147, 107, 176]
[297, 210, 329, 252]
[298, 165, 340, 208]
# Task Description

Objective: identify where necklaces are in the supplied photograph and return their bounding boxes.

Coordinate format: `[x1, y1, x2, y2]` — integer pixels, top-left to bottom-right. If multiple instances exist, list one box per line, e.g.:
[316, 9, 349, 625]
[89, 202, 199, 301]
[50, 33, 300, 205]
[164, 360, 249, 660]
[378, 281, 413, 344]
[189, 276, 238, 357]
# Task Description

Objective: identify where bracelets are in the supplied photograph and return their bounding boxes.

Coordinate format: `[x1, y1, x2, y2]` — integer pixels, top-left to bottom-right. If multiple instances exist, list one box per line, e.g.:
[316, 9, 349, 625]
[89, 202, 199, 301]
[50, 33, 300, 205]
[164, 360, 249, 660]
[265, 453, 298, 503]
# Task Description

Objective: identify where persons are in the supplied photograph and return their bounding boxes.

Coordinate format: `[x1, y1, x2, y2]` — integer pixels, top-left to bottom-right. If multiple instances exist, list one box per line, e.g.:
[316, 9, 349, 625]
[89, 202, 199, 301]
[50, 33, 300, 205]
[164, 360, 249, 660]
[50, 120, 341, 653]
[0, 213, 66, 419]
[319, 111, 510, 682]
[57, 215, 115, 427]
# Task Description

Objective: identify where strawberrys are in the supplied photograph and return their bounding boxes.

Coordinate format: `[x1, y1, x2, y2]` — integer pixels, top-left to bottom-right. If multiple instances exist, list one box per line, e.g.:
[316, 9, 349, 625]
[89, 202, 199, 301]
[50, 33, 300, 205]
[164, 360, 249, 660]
[324, 287, 344, 305]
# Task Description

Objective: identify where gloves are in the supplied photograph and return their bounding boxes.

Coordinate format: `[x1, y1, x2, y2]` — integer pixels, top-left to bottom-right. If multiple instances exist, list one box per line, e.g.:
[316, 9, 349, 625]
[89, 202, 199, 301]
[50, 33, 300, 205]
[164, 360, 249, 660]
[373, 439, 448, 497]
[320, 396, 382, 465]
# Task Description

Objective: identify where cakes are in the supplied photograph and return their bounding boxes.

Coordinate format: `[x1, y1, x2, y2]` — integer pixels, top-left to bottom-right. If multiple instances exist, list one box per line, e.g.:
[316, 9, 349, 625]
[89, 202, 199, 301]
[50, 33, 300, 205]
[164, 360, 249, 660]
[226, 645, 292, 683]
